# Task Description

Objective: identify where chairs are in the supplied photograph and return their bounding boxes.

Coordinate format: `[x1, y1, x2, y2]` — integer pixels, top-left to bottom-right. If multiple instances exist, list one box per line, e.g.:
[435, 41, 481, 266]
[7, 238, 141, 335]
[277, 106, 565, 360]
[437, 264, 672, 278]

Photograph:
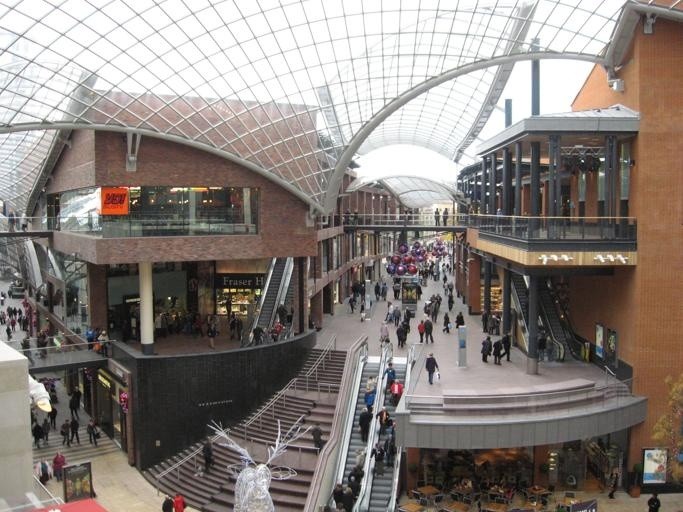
[396, 480, 575, 512]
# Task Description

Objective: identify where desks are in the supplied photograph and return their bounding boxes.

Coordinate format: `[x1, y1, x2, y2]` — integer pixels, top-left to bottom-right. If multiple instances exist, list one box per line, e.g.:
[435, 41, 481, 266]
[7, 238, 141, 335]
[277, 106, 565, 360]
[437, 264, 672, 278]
[554, 495, 582, 512]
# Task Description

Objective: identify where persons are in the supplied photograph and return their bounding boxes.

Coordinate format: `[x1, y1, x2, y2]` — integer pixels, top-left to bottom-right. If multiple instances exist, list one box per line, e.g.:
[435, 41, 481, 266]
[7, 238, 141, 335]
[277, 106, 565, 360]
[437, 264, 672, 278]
[435, 207, 441, 225]
[322, 237, 464, 512]
[0, 291, 101, 485]
[8, 208, 16, 232]
[121, 319, 131, 342]
[648, 491, 660, 512]
[21, 209, 31, 232]
[154, 292, 284, 350]
[312, 421, 323, 456]
[57, 211, 61, 230]
[162, 494, 174, 512]
[607, 472, 619, 500]
[203, 437, 214, 472]
[344, 209, 350, 224]
[537, 329, 553, 362]
[354, 209, 358, 226]
[482, 309, 510, 365]
[480, 475, 514, 499]
[174, 492, 186, 512]
[443, 208, 448, 225]
